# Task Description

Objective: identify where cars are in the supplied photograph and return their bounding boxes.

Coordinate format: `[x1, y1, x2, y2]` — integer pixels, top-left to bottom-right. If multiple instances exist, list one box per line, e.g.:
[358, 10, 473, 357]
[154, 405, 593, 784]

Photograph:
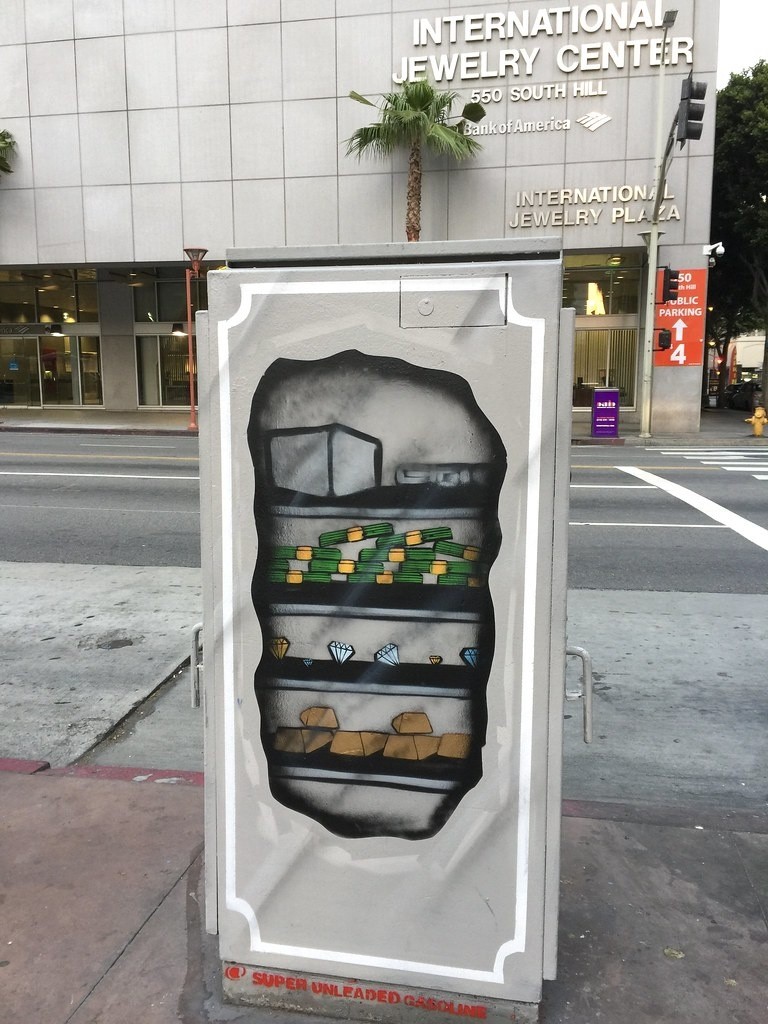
[732, 381, 763, 410]
[723, 383, 743, 407]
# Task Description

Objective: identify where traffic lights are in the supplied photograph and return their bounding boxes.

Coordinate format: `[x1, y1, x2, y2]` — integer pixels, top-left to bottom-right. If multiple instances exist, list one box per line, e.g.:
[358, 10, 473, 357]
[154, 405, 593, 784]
[660, 330, 671, 349]
[678, 75, 708, 151]
[664, 269, 679, 301]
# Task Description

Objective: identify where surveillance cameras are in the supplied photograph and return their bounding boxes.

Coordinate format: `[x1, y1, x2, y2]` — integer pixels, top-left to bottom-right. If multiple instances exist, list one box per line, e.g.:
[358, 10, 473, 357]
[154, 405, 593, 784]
[710, 258, 715, 267]
[716, 247, 725, 257]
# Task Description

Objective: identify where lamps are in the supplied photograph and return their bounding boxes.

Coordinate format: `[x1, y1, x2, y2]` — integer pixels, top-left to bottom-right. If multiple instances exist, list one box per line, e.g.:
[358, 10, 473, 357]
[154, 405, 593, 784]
[171, 323, 184, 334]
[50, 325, 61, 335]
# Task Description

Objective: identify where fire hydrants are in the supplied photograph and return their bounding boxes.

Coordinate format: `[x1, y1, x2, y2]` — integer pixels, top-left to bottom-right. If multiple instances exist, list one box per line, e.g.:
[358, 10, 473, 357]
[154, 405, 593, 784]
[745, 407, 768, 436]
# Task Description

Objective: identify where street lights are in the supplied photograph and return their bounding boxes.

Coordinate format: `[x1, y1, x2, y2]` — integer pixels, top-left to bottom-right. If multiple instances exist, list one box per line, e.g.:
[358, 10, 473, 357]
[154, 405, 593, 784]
[184, 247, 207, 311]
